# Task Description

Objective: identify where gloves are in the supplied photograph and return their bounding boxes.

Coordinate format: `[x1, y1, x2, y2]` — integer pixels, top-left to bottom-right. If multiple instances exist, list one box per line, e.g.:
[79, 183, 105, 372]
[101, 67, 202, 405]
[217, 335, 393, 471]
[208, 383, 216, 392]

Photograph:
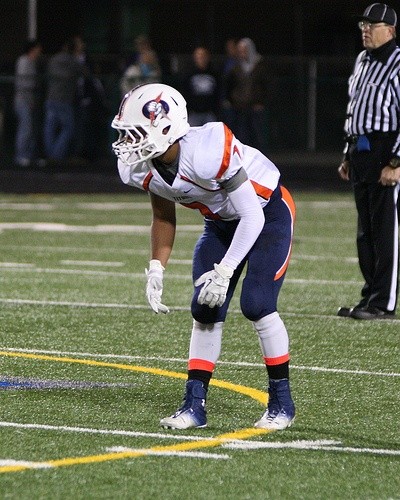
[145, 259, 170, 315]
[195, 264, 233, 308]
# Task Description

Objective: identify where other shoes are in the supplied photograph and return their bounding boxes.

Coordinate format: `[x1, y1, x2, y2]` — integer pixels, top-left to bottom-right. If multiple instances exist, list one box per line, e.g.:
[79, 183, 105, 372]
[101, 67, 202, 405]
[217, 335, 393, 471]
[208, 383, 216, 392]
[18, 159, 69, 170]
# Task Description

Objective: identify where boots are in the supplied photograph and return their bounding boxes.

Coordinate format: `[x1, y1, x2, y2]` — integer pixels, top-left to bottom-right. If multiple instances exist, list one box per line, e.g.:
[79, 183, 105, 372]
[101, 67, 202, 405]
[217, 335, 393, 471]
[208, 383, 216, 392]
[254, 380, 295, 430]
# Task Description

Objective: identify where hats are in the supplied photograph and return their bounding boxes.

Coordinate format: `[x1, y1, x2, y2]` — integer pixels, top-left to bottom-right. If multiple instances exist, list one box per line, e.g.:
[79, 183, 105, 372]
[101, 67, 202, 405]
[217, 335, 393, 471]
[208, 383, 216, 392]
[349, 3, 397, 26]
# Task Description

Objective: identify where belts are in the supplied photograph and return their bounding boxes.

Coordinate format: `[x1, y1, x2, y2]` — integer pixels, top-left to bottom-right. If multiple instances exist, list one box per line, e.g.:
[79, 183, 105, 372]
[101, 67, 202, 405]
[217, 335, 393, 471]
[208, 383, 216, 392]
[345, 135, 358, 144]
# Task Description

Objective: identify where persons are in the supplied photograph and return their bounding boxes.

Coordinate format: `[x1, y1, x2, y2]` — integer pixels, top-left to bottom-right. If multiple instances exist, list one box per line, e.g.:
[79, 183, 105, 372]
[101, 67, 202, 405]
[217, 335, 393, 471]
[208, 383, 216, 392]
[9, 30, 275, 176]
[335, 3, 400, 321]
[110, 83, 296, 431]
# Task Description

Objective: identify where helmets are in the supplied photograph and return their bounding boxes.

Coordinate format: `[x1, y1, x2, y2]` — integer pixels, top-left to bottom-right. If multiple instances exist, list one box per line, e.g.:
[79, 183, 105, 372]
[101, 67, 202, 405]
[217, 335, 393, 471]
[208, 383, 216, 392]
[111, 82, 190, 166]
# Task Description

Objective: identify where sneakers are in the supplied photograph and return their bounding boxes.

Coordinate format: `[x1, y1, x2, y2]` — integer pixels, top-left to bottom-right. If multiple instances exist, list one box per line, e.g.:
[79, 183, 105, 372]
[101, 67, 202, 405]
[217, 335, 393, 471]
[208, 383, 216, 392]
[336, 303, 395, 320]
[158, 380, 208, 430]
